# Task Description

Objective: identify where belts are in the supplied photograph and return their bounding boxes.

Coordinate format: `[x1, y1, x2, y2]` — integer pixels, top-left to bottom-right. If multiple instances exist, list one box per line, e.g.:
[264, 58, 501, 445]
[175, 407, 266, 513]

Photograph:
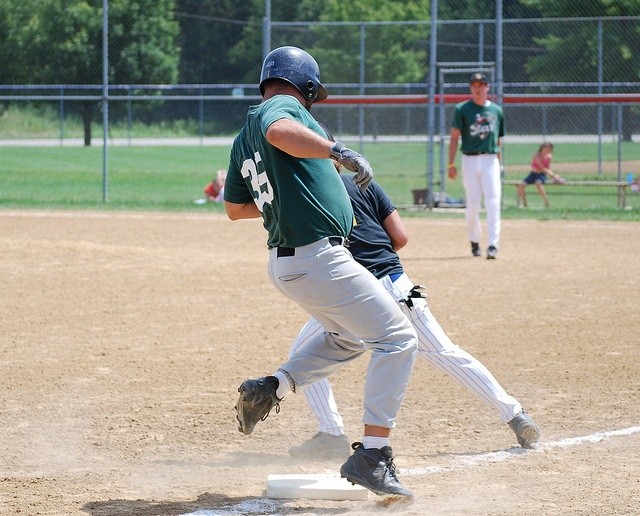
[277, 235, 348, 257]
[467, 151, 485, 155]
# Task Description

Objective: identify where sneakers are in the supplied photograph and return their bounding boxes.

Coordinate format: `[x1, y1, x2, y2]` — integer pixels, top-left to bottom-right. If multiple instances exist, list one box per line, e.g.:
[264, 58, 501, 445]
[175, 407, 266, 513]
[340, 442, 412, 502]
[487, 245, 496, 259]
[471, 241, 480, 256]
[508, 408, 539, 449]
[235, 376, 282, 435]
[288, 432, 350, 460]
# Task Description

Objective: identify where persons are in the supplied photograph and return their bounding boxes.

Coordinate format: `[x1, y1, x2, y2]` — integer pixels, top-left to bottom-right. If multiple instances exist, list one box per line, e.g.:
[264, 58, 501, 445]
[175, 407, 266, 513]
[519, 143, 561, 209]
[223, 46, 419, 501]
[448, 72, 506, 260]
[288, 122, 538, 460]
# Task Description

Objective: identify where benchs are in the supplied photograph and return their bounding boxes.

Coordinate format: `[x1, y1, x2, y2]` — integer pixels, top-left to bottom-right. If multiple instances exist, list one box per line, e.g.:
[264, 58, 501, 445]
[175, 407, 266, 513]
[501, 181, 635, 209]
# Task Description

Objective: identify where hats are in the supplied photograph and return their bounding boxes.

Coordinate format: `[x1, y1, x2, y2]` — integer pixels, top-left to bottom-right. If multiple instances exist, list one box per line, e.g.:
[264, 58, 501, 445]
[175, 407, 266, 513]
[469, 72, 488, 83]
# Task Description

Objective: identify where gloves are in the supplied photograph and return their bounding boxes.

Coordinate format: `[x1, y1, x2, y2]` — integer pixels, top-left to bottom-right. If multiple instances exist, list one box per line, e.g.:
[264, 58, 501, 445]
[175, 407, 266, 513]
[329, 141, 373, 191]
[399, 284, 427, 312]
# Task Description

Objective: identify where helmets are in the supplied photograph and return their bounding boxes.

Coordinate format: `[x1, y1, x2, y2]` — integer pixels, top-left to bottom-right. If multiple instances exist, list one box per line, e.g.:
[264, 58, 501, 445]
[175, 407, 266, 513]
[259, 46, 327, 113]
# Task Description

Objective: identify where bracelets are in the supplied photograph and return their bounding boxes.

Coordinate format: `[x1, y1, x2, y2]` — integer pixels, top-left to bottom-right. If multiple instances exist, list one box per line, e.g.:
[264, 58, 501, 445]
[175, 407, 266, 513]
[447, 163, 456, 169]
